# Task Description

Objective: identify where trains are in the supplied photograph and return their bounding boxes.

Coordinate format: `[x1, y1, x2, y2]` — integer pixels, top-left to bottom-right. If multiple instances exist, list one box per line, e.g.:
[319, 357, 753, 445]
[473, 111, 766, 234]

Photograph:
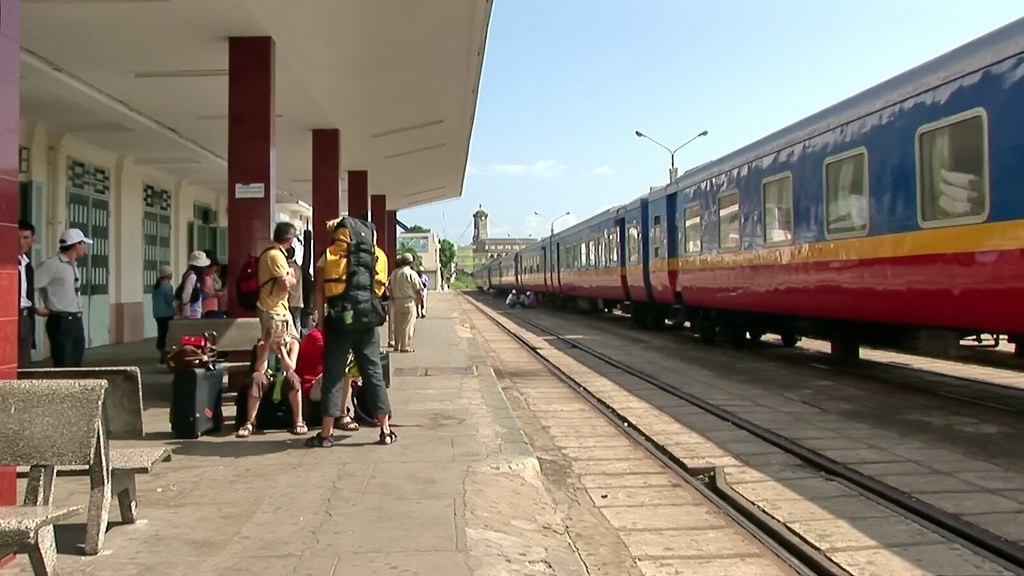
[473, 15, 1024, 372]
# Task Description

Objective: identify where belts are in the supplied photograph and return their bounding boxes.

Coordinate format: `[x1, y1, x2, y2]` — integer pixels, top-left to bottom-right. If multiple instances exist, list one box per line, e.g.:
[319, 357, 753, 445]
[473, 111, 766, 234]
[56, 313, 82, 318]
[20, 309, 28, 316]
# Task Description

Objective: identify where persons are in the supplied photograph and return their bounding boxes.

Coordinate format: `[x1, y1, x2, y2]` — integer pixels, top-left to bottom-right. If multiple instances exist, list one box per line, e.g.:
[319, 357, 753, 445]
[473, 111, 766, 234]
[305, 214, 398, 447]
[18, 219, 37, 368]
[152, 223, 428, 430]
[34, 228, 93, 366]
[505, 289, 538, 309]
[235, 309, 308, 438]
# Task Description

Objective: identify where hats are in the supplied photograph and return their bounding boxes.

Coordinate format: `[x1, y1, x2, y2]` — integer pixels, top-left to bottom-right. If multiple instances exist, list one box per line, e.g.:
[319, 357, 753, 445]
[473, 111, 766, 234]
[59, 228, 94, 246]
[402, 253, 413, 262]
[527, 291, 531, 296]
[512, 289, 517, 294]
[188, 251, 211, 267]
[159, 265, 174, 276]
[417, 266, 425, 272]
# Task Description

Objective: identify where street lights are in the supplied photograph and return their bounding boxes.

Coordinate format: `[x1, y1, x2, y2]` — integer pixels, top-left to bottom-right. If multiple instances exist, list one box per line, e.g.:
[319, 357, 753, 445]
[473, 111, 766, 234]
[535, 211, 570, 234]
[635, 131, 709, 168]
[508, 234, 533, 249]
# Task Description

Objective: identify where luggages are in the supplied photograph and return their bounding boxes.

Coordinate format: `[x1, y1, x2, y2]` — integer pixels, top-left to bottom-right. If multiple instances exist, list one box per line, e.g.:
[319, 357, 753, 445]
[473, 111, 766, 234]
[170, 331, 224, 438]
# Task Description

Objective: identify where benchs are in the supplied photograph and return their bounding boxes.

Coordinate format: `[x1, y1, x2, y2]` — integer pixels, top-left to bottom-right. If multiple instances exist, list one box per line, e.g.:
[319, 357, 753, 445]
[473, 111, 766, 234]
[163, 318, 262, 403]
[16, 367, 173, 557]
[0, 379, 111, 576]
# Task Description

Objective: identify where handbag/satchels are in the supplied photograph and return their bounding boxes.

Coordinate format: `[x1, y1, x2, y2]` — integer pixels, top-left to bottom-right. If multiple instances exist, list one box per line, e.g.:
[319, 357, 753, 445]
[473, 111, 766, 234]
[173, 281, 200, 304]
[166, 345, 217, 369]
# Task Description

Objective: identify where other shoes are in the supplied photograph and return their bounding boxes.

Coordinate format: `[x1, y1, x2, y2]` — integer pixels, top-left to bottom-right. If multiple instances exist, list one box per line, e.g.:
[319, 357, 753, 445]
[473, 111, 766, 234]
[294, 420, 308, 434]
[237, 422, 257, 436]
[388, 341, 394, 347]
[400, 348, 414, 352]
[394, 348, 400, 351]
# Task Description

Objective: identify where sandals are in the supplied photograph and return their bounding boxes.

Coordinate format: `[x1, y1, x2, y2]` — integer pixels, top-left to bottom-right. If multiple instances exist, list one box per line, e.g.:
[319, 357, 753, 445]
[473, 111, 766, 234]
[305, 432, 334, 448]
[382, 430, 397, 445]
[334, 414, 360, 431]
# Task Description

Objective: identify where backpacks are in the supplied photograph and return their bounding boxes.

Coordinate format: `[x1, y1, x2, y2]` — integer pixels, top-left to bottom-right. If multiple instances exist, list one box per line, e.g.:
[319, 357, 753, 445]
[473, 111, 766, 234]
[323, 217, 387, 328]
[351, 376, 391, 426]
[236, 246, 281, 311]
